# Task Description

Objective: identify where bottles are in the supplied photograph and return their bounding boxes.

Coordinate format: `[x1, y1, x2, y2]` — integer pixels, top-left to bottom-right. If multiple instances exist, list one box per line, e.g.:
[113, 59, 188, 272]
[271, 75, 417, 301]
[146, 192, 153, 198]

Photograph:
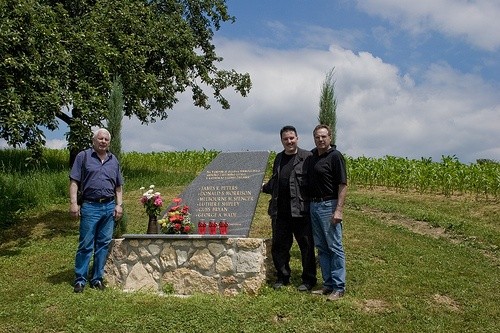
[219, 219, 228, 235]
[208, 219, 218, 235]
[198, 218, 206, 235]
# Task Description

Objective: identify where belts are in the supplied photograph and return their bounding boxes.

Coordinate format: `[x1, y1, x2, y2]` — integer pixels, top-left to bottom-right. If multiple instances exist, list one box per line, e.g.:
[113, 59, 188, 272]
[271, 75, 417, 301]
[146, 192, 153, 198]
[83, 196, 115, 204]
[310, 196, 337, 203]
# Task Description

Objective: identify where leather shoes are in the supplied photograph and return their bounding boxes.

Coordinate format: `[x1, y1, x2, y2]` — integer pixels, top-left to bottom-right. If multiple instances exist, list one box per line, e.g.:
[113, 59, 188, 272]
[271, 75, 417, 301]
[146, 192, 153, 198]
[297, 280, 317, 290]
[75, 283, 84, 293]
[89, 280, 107, 291]
[273, 279, 290, 289]
[311, 287, 332, 295]
[326, 290, 344, 301]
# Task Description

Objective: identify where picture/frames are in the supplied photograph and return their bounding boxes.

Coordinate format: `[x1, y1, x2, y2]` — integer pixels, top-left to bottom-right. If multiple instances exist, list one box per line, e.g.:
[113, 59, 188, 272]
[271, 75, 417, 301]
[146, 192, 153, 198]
[147, 214, 158, 234]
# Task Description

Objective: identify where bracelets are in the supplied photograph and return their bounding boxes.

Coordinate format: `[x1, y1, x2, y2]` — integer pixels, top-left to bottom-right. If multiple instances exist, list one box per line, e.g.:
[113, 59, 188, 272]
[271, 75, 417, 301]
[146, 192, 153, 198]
[116, 204, 124, 207]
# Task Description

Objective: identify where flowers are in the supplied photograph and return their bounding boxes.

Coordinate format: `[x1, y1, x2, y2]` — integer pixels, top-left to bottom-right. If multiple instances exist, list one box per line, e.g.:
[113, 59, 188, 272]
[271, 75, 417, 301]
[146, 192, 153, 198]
[140, 185, 163, 217]
[157, 198, 196, 234]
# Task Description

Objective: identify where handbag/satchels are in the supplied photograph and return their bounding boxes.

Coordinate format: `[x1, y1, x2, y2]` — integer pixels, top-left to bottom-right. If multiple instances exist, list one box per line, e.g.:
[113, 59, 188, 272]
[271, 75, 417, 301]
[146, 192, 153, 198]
[77, 191, 83, 205]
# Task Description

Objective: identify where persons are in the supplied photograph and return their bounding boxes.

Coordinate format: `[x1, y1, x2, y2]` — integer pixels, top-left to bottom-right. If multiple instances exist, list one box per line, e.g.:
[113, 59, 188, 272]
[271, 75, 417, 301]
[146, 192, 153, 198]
[69, 128, 125, 293]
[260, 126, 317, 291]
[309, 125, 348, 301]
